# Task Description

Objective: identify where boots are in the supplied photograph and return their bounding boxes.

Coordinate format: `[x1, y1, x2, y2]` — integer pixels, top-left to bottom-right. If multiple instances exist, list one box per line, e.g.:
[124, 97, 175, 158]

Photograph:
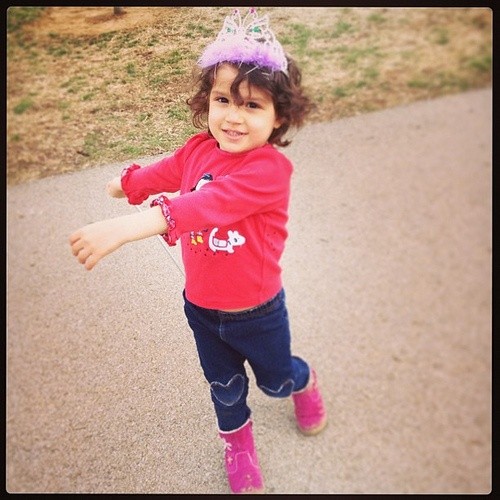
[290, 356, 327, 434]
[216, 416, 264, 493]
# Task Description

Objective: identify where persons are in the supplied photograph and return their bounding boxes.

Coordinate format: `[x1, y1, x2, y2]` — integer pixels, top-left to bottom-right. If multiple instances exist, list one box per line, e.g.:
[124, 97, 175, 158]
[67, 33, 329, 495]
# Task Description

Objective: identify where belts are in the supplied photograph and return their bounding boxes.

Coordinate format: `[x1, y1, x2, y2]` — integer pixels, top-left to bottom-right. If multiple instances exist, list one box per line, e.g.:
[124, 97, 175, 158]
[183, 289, 286, 323]
